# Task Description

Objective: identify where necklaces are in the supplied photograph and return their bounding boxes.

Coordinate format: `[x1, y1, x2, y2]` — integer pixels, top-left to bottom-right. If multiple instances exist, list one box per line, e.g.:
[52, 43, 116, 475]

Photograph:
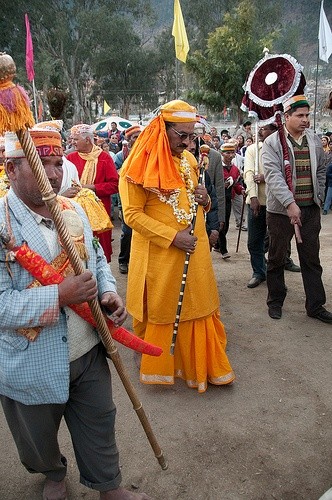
[165, 152, 194, 226]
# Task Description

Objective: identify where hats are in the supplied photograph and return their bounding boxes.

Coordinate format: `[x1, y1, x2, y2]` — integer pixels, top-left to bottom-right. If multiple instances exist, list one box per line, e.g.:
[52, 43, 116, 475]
[221, 143, 236, 151]
[125, 125, 141, 136]
[71, 125, 91, 134]
[194, 123, 205, 136]
[4, 120, 63, 158]
[283, 95, 309, 113]
[161, 100, 197, 122]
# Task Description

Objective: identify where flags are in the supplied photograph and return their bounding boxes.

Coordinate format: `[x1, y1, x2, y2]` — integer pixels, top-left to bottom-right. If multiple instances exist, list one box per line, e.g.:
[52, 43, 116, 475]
[317, 0, 332, 64]
[24, 13, 35, 82]
[171, 0, 191, 63]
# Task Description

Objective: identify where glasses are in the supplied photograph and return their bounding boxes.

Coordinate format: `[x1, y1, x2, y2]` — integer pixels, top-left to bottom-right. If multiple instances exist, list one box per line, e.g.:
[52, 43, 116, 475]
[167, 126, 196, 141]
[261, 128, 272, 131]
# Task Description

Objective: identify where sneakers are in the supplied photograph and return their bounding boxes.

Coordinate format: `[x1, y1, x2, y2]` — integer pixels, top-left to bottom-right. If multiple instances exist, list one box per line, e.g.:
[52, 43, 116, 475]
[214, 248, 220, 252]
[247, 277, 265, 288]
[222, 253, 231, 259]
[268, 306, 282, 319]
[310, 308, 332, 323]
[119, 263, 129, 273]
[285, 263, 300, 272]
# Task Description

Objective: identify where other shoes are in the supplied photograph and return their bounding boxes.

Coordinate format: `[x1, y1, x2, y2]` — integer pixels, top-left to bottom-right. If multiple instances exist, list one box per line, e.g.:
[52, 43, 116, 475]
[236, 224, 247, 230]
[323, 210, 327, 215]
[42, 477, 67, 500]
[100, 486, 151, 500]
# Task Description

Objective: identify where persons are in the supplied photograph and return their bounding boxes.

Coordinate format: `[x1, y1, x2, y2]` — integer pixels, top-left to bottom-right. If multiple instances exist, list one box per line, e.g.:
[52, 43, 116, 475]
[261, 91, 332, 324]
[117, 100, 235, 394]
[314, 91, 332, 215]
[58, 120, 141, 275]
[0, 118, 152, 499]
[186, 112, 302, 288]
